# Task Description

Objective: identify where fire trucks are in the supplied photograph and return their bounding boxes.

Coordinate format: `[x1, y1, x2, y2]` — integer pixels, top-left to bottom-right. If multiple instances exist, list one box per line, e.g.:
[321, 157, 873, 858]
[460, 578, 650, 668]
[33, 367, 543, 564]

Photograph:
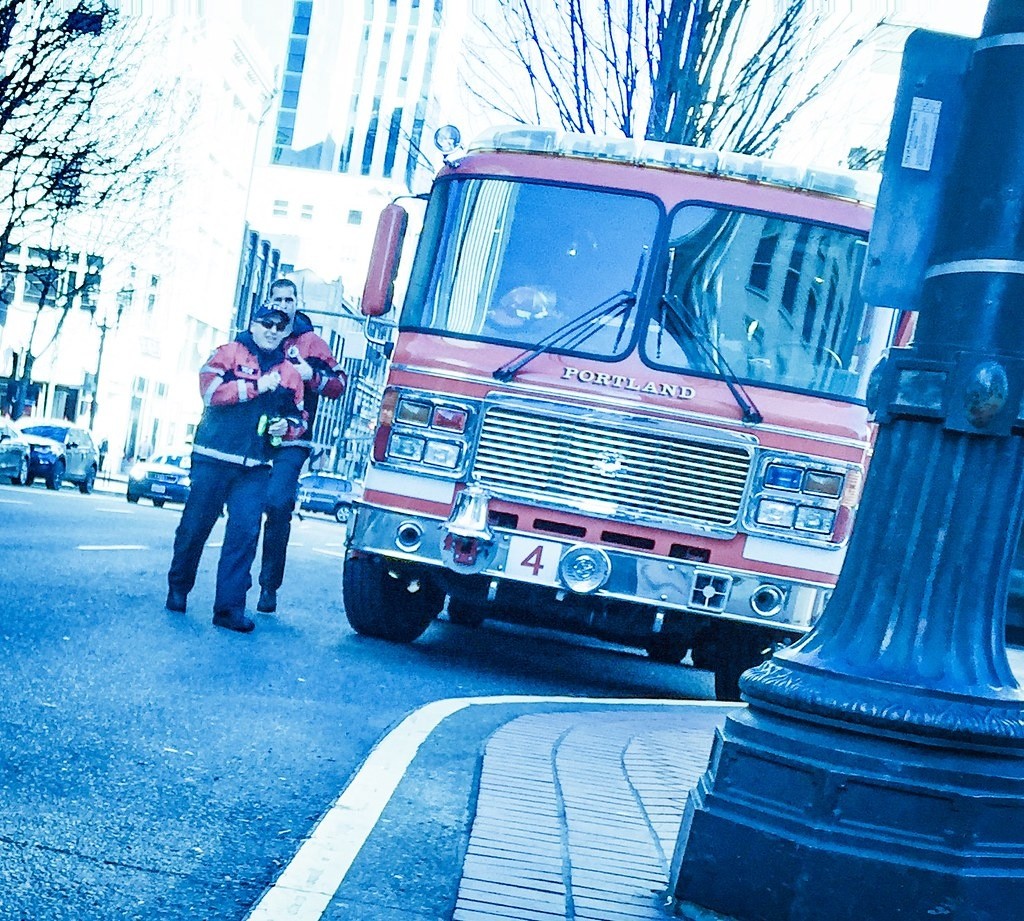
[343, 124, 917, 702]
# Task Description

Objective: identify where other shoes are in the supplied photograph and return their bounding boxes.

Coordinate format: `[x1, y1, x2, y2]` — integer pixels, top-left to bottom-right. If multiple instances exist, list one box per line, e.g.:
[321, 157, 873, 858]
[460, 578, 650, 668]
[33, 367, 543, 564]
[166, 588, 187, 613]
[257, 588, 276, 613]
[212, 612, 255, 632]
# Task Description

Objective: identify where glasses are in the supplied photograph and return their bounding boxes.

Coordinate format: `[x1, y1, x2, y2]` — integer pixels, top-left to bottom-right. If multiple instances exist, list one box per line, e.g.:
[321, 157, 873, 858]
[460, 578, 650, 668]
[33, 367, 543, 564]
[254, 320, 286, 331]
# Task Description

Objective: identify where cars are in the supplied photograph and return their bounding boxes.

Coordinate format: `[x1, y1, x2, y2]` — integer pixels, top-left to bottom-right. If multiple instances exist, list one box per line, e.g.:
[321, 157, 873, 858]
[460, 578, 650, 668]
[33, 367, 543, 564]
[17, 420, 100, 495]
[0, 417, 31, 486]
[127, 448, 191, 507]
[298, 472, 364, 524]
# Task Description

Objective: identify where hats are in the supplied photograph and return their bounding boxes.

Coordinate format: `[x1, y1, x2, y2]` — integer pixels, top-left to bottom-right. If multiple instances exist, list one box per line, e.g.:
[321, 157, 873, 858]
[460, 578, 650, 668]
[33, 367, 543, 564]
[255, 303, 290, 325]
[486, 286, 549, 328]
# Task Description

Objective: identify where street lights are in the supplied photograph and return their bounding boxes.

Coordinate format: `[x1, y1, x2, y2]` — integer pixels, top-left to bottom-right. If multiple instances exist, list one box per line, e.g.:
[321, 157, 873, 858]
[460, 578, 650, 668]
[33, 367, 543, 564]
[89, 300, 124, 431]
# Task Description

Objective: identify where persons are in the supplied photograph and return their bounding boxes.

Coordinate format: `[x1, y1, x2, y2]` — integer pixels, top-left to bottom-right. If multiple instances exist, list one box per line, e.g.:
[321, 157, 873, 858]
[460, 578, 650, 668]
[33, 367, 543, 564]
[165, 279, 345, 633]
